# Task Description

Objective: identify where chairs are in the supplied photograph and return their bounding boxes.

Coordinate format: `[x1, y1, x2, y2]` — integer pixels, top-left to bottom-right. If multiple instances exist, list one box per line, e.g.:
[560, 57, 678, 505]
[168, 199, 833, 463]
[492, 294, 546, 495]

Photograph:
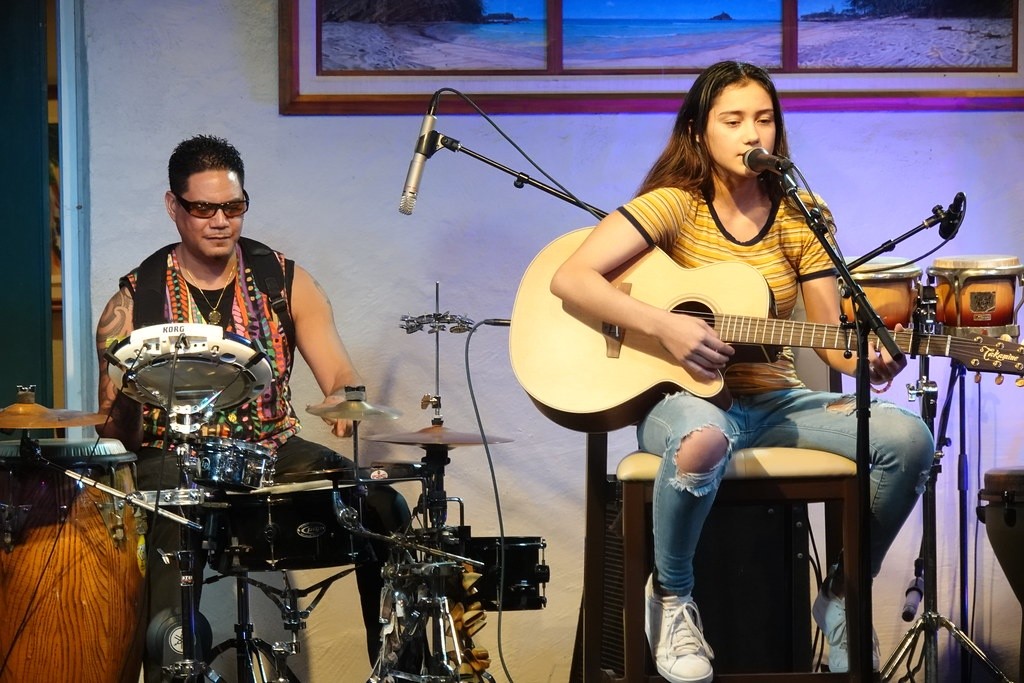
[583, 282, 873, 683]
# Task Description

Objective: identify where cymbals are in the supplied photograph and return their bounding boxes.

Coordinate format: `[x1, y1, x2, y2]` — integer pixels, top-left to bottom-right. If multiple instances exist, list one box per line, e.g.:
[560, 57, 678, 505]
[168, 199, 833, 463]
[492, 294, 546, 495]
[0, 402, 113, 429]
[305, 400, 404, 422]
[358, 426, 513, 450]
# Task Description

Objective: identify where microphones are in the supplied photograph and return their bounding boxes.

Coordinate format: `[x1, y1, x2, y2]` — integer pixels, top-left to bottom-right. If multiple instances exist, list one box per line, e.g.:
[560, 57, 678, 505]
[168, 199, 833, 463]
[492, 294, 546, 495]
[398, 89, 441, 214]
[742, 147, 794, 173]
[940, 191, 965, 240]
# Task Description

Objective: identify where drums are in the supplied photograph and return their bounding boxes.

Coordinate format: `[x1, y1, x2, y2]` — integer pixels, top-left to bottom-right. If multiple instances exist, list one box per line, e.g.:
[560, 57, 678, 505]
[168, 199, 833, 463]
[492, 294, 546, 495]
[0, 438, 150, 683]
[933, 255, 1019, 338]
[837, 256, 924, 330]
[206, 479, 359, 574]
[191, 435, 277, 489]
[138, 488, 213, 581]
[423, 535, 543, 612]
[106, 322, 277, 414]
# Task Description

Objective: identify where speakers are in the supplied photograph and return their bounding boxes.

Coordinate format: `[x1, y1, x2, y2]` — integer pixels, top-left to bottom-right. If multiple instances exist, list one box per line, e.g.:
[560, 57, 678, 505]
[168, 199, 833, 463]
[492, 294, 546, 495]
[593, 466, 810, 676]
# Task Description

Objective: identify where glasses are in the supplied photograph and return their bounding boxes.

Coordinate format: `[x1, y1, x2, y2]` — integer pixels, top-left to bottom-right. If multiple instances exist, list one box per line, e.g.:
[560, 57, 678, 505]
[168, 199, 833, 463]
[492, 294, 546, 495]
[176, 188, 250, 218]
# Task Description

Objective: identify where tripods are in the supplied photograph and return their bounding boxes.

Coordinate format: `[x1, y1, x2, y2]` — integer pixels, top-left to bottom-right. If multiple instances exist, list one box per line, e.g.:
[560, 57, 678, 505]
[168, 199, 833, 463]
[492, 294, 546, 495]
[878, 353, 1011, 683]
[156, 445, 496, 683]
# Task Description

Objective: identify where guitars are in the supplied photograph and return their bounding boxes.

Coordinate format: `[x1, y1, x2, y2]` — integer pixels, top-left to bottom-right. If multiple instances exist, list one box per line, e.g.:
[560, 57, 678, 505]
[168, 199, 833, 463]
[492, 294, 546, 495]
[508, 225, 1024, 435]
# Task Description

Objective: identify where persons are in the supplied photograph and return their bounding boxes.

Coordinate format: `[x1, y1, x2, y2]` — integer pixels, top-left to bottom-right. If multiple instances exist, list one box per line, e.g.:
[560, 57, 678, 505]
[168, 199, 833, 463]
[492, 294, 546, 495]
[96, 133, 434, 683]
[549, 57, 935, 683]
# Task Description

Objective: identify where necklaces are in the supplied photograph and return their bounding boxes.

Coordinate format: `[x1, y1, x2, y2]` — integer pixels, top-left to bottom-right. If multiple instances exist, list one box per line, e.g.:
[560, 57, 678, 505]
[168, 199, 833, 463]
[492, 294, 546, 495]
[180, 252, 236, 324]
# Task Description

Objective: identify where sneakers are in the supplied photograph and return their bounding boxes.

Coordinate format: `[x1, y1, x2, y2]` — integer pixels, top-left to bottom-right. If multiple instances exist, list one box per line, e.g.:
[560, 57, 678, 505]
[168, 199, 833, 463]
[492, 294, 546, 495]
[643, 575, 714, 683]
[812, 591, 881, 672]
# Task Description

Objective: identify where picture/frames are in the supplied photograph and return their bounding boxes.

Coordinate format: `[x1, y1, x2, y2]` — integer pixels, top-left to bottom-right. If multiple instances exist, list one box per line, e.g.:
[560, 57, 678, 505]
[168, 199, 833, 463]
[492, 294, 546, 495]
[278, 0, 1023, 116]
